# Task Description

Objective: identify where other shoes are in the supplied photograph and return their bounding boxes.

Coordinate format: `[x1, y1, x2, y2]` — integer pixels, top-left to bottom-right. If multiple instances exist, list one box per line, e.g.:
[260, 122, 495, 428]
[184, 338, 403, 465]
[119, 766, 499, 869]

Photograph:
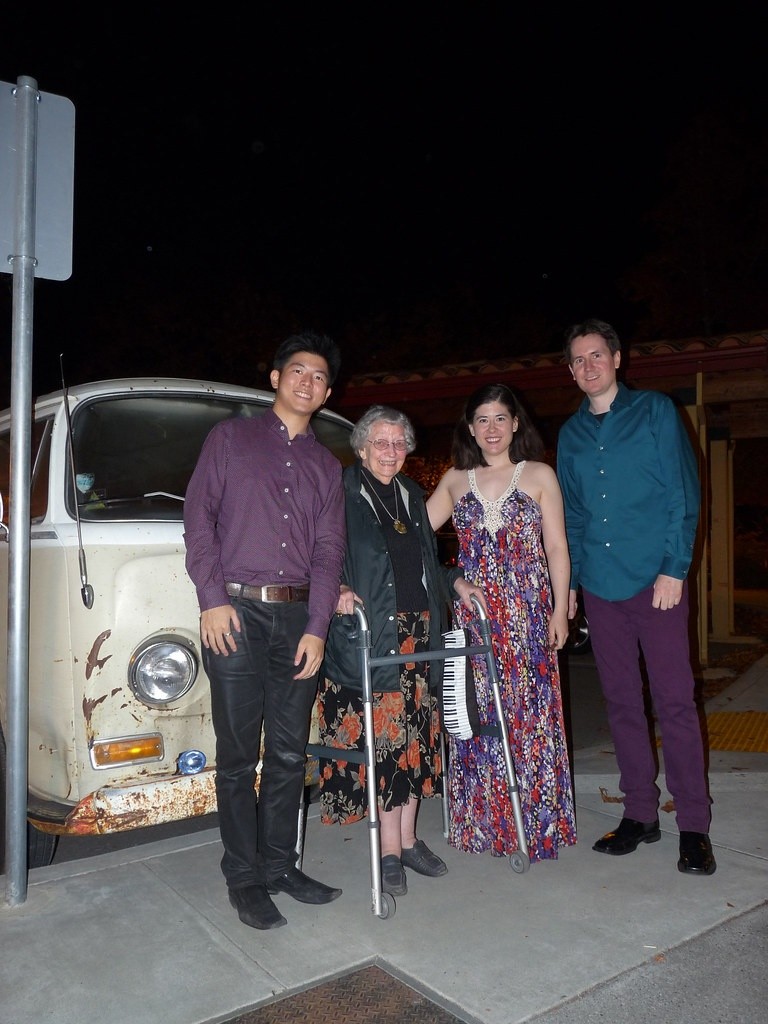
[400, 839, 447, 878]
[381, 854, 407, 896]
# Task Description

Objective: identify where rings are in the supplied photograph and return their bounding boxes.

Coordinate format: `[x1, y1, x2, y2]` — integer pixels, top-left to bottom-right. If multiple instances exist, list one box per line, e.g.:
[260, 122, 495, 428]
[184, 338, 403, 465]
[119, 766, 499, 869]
[225, 632, 232, 636]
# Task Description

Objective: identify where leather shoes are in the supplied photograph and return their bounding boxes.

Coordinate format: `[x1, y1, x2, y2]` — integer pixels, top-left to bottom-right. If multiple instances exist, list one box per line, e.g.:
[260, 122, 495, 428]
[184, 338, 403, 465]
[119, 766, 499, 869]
[266, 865, 343, 905]
[677, 831, 717, 875]
[591, 816, 661, 856]
[228, 882, 287, 930]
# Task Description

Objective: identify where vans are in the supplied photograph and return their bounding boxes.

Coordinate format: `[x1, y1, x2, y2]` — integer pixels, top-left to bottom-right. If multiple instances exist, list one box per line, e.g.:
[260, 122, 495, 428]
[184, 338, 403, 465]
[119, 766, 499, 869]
[1, 375, 359, 876]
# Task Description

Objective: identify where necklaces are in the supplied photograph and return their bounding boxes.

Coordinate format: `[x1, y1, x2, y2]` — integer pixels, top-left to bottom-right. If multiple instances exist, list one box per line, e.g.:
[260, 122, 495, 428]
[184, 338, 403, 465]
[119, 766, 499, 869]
[362, 466, 406, 536]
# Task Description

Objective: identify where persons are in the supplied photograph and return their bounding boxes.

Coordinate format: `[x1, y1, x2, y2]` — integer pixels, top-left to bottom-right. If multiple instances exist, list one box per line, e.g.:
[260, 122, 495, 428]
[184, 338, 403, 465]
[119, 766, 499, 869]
[181, 334, 341, 930]
[425, 380, 576, 865]
[326, 405, 448, 894]
[558, 319, 717, 876]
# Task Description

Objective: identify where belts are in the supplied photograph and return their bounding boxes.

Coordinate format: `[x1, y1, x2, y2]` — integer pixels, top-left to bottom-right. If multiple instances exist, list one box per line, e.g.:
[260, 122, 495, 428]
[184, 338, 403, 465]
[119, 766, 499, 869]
[224, 582, 309, 604]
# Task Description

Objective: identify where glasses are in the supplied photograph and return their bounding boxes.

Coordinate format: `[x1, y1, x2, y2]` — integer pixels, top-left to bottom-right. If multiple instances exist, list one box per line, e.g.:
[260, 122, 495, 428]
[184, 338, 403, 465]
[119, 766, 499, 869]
[367, 438, 411, 451]
